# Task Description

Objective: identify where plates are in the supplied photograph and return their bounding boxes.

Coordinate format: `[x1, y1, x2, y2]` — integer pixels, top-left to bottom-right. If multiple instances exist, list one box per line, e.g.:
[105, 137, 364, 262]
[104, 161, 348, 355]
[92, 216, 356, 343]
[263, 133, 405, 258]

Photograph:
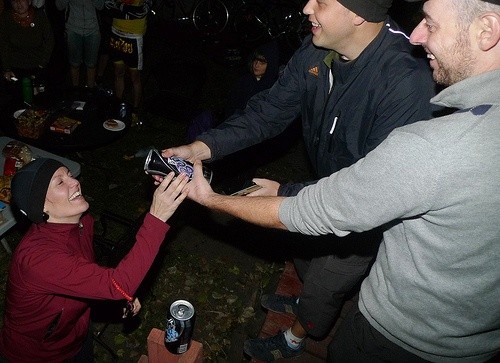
[13, 109, 25, 118]
[103, 119, 126, 131]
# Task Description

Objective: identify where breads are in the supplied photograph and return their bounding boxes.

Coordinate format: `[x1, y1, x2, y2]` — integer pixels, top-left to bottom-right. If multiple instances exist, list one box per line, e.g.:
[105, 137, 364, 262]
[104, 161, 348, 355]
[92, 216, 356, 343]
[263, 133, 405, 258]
[3, 141, 33, 164]
[105, 120, 118, 129]
[17, 110, 50, 139]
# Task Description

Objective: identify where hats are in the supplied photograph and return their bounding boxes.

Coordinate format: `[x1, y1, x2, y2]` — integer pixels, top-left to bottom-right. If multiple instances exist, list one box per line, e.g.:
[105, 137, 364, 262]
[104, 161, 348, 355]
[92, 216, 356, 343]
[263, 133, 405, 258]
[337, 0, 392, 24]
[252, 39, 278, 63]
[10, 157, 70, 223]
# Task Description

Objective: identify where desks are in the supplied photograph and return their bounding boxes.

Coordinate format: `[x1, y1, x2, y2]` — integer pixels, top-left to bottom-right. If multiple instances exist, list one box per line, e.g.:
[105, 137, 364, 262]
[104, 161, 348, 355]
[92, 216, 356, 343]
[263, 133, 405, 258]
[0, 136, 83, 255]
[12, 84, 133, 167]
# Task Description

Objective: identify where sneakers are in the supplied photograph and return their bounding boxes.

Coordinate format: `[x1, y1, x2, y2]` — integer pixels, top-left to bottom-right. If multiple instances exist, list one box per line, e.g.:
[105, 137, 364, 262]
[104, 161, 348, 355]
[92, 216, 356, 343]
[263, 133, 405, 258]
[261, 294, 299, 318]
[243, 330, 307, 362]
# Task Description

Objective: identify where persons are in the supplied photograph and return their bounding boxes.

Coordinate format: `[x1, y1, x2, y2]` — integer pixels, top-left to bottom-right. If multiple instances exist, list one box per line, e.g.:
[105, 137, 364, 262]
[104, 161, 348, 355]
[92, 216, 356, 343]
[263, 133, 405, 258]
[180, 0, 500, 363]
[0, 156, 190, 363]
[234, 46, 274, 114]
[105, 0, 153, 128]
[55, 0, 104, 90]
[0, 0, 53, 81]
[152, 0, 450, 360]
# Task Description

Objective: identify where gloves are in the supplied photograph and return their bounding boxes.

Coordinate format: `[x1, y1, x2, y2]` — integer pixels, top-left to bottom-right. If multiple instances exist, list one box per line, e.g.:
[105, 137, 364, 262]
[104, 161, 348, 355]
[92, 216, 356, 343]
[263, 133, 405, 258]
[104, 0, 125, 12]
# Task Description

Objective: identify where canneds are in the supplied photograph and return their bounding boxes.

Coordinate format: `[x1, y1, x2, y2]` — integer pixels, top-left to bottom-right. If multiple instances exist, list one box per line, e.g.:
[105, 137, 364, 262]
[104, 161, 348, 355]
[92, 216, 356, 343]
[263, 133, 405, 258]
[143, 148, 213, 185]
[164, 300, 195, 355]
[120, 103, 127, 119]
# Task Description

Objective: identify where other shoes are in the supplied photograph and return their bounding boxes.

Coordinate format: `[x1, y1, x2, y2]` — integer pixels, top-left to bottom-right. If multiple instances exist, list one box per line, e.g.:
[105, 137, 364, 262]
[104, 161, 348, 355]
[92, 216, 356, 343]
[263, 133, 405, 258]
[130, 113, 139, 127]
